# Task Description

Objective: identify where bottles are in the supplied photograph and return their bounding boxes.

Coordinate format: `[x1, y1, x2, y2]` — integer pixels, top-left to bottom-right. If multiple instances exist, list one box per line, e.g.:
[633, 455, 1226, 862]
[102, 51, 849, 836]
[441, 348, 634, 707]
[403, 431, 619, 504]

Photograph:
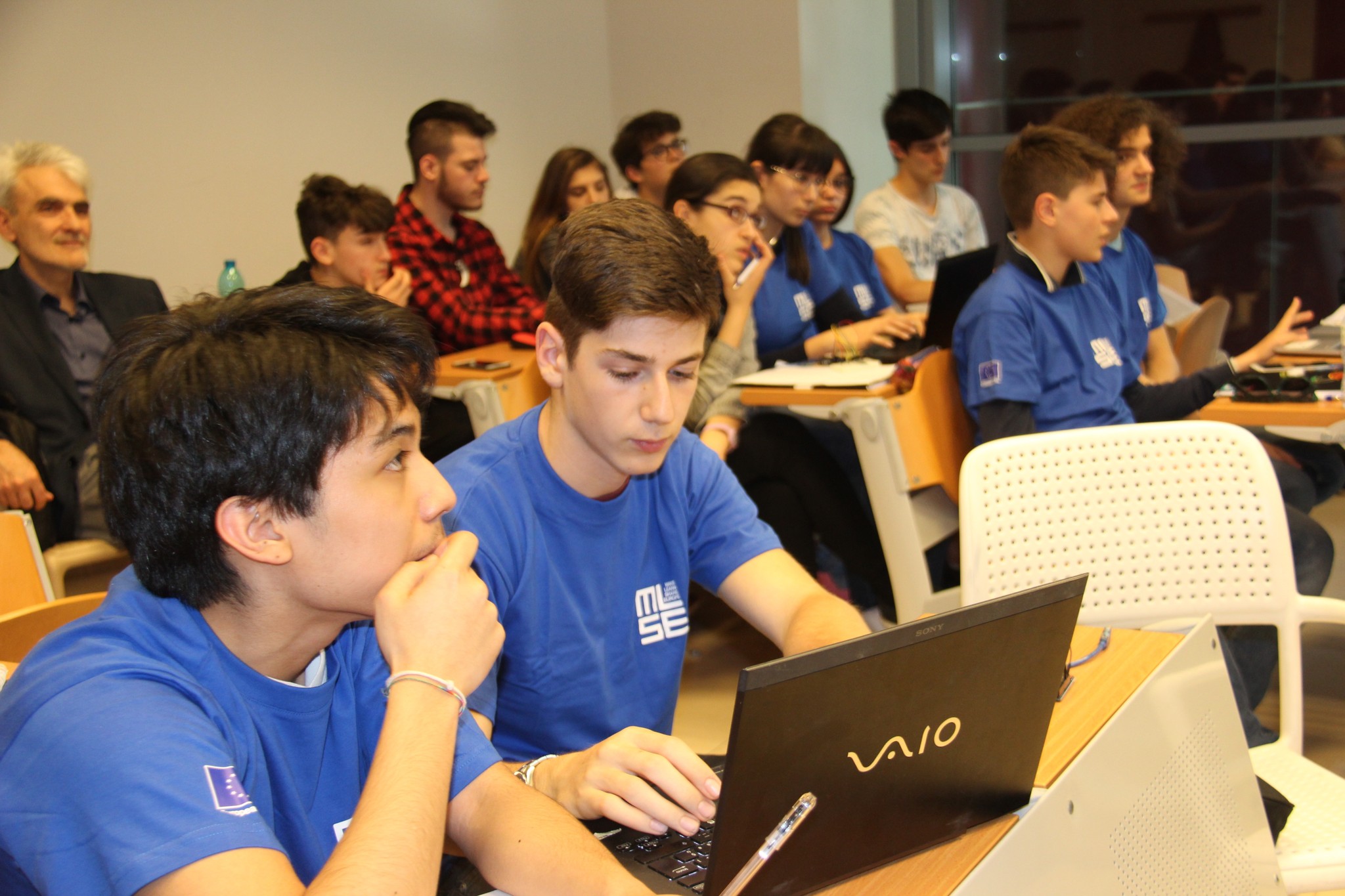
[218, 260, 244, 297]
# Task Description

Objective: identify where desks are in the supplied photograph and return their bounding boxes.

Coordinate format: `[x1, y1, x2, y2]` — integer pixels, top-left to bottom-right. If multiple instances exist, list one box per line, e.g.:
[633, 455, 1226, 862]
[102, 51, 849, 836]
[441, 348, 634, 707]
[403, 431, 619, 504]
[0, 268, 1344, 896]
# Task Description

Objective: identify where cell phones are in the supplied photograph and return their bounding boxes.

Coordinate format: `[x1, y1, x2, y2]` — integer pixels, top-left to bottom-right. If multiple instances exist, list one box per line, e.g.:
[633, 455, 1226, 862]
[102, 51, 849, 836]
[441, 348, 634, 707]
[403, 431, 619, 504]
[451, 360, 512, 370]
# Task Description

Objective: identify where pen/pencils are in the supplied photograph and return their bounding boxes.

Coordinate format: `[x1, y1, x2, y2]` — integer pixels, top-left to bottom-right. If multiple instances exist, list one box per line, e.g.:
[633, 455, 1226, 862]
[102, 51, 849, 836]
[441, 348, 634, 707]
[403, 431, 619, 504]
[732, 238, 777, 291]
[719, 792, 817, 896]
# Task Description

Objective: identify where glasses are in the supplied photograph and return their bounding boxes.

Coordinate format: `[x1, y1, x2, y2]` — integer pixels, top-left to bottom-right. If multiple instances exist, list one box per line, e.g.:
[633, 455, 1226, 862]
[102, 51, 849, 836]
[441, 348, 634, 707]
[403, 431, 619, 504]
[699, 198, 767, 229]
[773, 163, 831, 195]
[646, 138, 690, 159]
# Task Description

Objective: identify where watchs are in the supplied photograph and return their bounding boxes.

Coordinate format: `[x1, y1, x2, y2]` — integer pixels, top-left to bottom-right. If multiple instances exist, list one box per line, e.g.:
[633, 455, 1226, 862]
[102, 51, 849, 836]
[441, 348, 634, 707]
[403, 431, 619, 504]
[515, 754, 560, 789]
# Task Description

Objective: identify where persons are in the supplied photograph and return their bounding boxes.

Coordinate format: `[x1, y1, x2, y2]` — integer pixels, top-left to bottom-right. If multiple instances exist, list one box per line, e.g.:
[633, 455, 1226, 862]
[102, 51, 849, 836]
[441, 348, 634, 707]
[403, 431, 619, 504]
[434, 198, 873, 835]
[0, 279, 653, 896]
[0, 142, 170, 543]
[264, 88, 1336, 739]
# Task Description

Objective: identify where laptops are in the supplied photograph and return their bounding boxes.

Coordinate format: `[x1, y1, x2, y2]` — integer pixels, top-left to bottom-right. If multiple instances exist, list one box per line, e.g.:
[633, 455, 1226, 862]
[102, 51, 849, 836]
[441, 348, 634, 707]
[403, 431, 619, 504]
[585, 572, 1089, 896]
[862, 243, 997, 366]
[1275, 325, 1343, 357]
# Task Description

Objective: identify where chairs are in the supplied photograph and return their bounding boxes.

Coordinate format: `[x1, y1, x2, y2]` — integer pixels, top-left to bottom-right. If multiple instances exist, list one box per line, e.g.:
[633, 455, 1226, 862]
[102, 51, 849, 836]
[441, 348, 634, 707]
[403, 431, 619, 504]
[957, 418, 1345, 804]
[725, 396, 964, 630]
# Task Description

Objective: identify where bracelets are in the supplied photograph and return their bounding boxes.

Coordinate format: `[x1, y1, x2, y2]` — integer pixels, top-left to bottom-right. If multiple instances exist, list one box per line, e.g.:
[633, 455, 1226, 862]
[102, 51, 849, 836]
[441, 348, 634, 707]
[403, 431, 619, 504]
[383, 670, 466, 716]
[385, 678, 464, 717]
